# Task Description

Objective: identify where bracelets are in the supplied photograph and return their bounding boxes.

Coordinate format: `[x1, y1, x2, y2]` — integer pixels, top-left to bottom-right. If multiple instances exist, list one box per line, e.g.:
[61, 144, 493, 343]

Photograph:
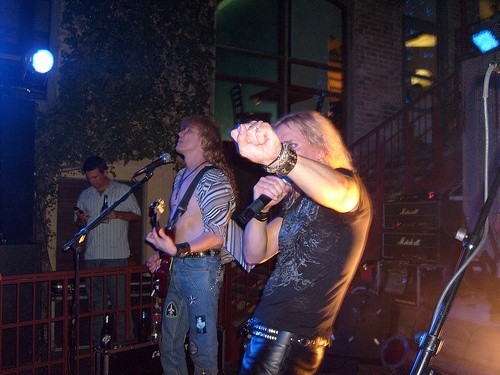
[263, 141, 297, 178]
[254, 211, 269, 222]
[173, 242, 191, 258]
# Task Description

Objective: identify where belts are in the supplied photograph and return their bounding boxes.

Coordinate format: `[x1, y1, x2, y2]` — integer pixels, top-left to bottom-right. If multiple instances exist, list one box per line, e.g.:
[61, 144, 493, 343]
[184, 249, 221, 258]
[242, 319, 330, 350]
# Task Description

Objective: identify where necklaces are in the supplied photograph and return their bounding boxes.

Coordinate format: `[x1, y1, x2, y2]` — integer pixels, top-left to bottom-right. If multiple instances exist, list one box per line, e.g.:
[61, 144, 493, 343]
[173, 161, 208, 205]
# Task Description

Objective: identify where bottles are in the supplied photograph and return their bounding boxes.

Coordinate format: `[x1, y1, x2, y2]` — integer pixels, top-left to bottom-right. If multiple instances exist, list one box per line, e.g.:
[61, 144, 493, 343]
[100, 315, 112, 350]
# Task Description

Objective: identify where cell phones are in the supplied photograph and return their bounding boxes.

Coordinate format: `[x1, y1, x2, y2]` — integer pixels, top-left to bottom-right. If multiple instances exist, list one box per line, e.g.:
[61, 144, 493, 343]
[73, 207, 84, 214]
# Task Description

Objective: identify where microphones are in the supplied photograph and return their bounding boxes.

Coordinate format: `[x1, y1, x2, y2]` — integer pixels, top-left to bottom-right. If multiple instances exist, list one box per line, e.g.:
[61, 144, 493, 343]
[489, 61, 500, 75]
[133, 152, 172, 177]
[238, 176, 291, 225]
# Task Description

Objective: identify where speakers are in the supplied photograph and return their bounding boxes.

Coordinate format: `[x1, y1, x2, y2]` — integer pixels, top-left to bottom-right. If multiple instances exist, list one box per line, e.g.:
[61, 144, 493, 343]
[317, 262, 439, 375]
[43, 295, 90, 351]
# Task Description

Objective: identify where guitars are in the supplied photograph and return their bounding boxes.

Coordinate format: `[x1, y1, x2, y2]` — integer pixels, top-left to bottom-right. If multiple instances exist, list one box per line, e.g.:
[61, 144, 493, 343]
[148, 199, 177, 295]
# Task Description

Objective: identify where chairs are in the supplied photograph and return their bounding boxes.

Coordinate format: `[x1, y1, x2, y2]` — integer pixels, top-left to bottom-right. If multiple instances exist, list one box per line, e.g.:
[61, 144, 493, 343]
[229, 83, 272, 128]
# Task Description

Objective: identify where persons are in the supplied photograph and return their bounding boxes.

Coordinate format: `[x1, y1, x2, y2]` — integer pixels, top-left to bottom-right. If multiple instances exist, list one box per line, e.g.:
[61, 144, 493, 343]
[146, 118, 238, 375]
[72, 156, 142, 353]
[231, 111, 372, 375]
